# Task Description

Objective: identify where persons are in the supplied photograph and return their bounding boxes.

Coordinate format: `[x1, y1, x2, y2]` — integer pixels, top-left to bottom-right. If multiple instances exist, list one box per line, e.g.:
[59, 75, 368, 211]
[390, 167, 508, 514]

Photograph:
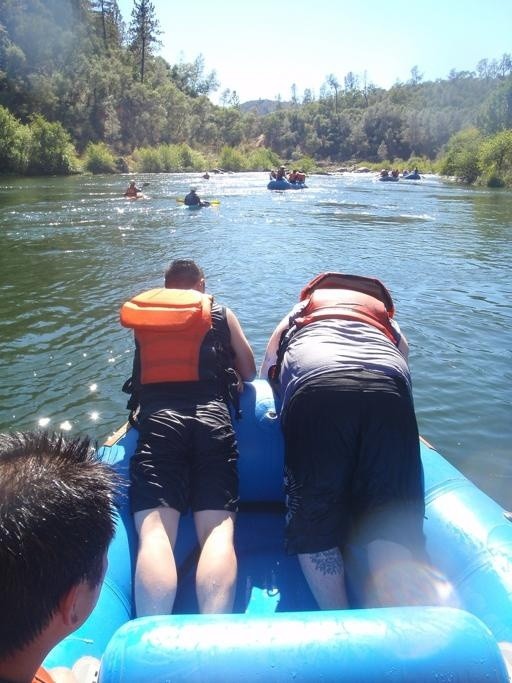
[270, 166, 305, 184]
[125, 181, 140, 196]
[414, 168, 419, 175]
[185, 186, 201, 205]
[267, 271, 424, 610]
[381, 169, 400, 178]
[403, 168, 409, 176]
[0, 430, 115, 683]
[203, 172, 209, 179]
[128, 260, 256, 614]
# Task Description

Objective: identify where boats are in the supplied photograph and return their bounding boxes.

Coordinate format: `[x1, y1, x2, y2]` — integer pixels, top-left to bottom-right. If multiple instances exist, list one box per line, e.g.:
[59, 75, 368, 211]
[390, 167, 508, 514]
[268, 176, 305, 189]
[379, 175, 400, 181]
[39, 376, 510, 683]
[404, 173, 420, 178]
[182, 198, 203, 209]
[126, 191, 143, 199]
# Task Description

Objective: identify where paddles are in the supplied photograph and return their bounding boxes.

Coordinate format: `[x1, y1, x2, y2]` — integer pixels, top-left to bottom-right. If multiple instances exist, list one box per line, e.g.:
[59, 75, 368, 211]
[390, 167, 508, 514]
[175, 198, 220, 206]
